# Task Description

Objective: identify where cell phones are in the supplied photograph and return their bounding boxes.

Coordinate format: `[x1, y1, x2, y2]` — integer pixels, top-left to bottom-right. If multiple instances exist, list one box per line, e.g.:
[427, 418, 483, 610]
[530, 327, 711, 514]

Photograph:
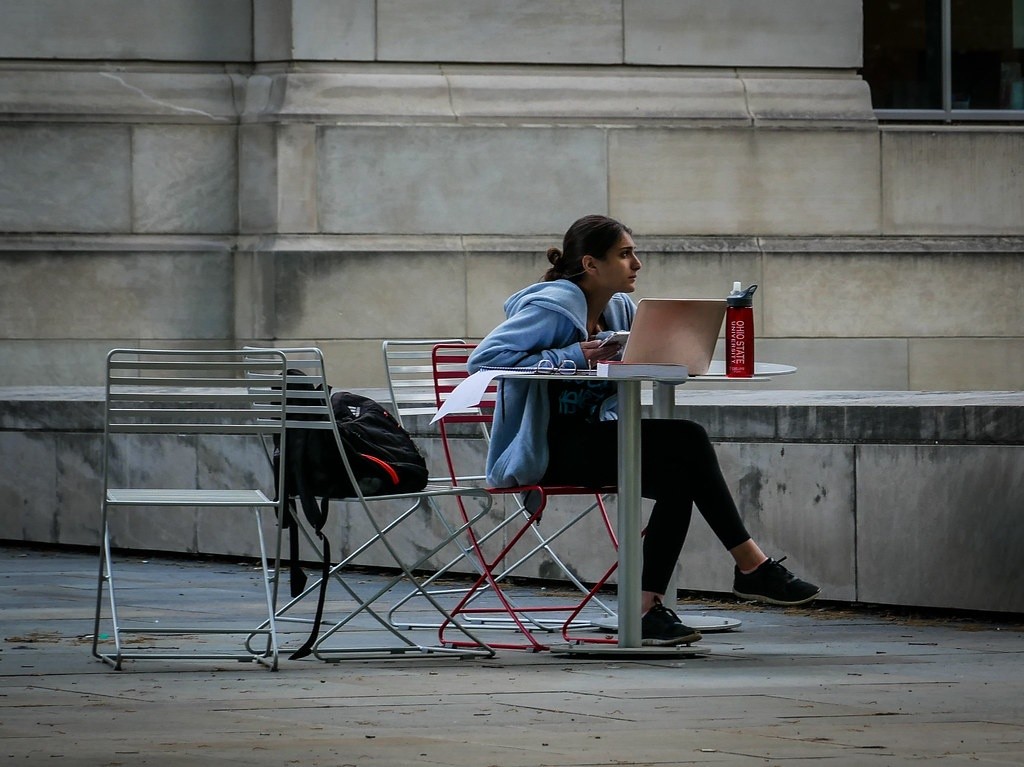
[598, 332, 631, 348]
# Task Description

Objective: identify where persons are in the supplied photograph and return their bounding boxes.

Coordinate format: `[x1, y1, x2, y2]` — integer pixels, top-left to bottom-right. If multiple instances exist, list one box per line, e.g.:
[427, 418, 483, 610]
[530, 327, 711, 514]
[466, 215, 823, 646]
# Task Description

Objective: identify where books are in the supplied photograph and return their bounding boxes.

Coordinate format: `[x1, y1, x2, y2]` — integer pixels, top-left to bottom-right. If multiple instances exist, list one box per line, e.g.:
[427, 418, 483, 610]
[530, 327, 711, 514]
[597, 360, 689, 380]
[429, 365, 597, 426]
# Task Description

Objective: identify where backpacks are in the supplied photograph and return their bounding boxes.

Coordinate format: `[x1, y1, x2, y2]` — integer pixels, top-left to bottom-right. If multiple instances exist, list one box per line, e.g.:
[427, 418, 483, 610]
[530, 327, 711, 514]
[269, 368, 428, 660]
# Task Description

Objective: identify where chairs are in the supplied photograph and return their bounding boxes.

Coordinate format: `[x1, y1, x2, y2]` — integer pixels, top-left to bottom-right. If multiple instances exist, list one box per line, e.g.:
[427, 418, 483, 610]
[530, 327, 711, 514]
[91, 347, 288, 673]
[241, 339, 648, 663]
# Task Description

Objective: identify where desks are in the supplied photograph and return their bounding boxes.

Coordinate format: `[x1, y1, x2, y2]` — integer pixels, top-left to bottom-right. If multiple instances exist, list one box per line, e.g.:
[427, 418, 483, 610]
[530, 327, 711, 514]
[482, 369, 771, 662]
[590, 360, 797, 633]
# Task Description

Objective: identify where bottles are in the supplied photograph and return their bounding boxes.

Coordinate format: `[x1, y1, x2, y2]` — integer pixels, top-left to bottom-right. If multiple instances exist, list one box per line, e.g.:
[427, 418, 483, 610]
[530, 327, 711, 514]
[726, 282, 757, 377]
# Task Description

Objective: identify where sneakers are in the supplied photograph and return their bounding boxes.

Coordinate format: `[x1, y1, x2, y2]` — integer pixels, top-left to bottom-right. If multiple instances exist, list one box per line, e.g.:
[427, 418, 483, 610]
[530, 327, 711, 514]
[732, 556, 821, 606]
[637, 595, 702, 646]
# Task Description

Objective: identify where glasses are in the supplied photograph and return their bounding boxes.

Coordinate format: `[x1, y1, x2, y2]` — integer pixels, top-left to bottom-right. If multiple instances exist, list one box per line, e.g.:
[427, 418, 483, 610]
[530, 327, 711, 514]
[535, 359, 578, 376]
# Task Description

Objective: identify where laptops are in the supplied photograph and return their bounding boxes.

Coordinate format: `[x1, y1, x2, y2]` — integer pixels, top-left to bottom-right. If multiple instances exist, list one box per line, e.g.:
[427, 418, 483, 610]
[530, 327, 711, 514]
[621, 299, 727, 376]
